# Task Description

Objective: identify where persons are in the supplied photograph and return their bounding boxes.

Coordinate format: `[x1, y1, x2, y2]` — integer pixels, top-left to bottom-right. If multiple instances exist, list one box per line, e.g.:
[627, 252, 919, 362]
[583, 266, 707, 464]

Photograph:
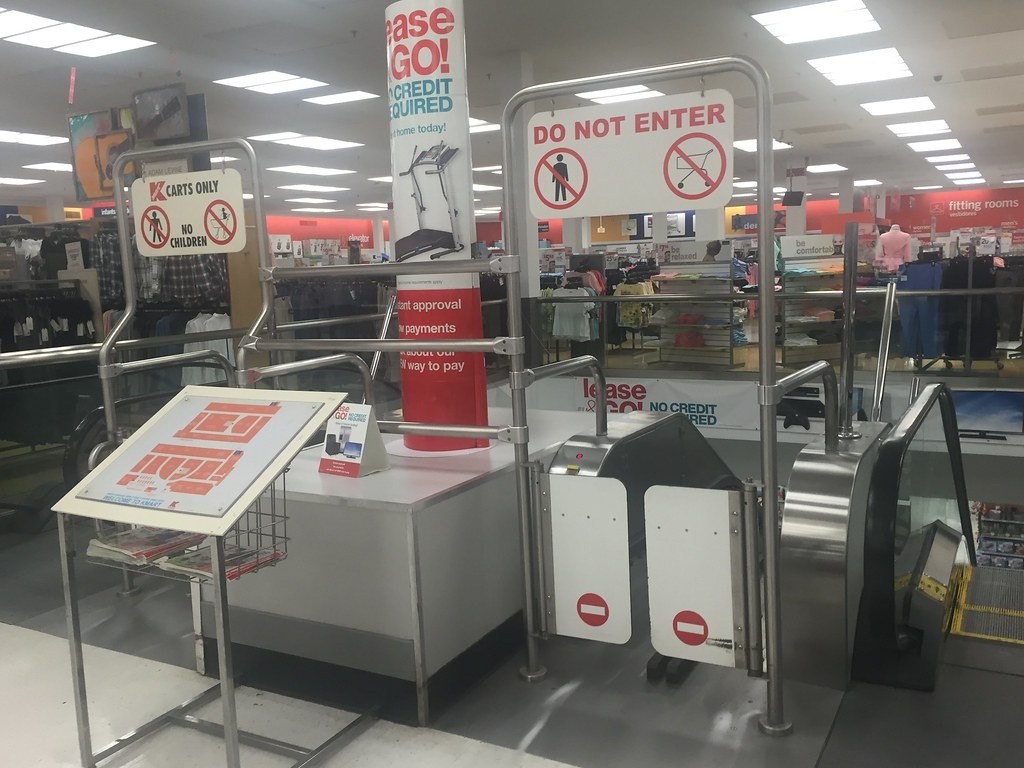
[880, 224, 911, 271]
[703, 241, 721, 261]
[734, 214, 743, 232]
[830, 238, 844, 255]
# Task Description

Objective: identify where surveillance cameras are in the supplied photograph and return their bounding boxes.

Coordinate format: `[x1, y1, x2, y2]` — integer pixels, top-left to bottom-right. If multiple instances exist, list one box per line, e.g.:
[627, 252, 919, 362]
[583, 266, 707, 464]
[933, 75, 942, 82]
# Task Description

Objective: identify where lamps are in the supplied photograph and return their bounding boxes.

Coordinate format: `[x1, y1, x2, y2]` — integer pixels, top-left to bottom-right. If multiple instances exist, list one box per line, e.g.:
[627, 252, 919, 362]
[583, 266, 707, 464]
[597, 216, 605, 233]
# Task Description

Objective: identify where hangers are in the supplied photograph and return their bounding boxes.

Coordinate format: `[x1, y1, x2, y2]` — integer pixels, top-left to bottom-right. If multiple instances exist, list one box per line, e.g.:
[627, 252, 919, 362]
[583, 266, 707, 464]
[562, 278, 584, 289]
[904, 248, 966, 265]
[14, 222, 118, 239]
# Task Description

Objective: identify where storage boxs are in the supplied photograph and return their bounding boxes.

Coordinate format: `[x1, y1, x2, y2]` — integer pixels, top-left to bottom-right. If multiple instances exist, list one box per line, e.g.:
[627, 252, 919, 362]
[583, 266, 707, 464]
[292, 241, 303, 259]
[269, 234, 292, 253]
[305, 239, 341, 257]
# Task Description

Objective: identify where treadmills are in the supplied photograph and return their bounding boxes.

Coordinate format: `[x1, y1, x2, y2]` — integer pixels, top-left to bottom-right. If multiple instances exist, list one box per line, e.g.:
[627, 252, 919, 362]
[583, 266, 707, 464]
[395, 139, 466, 262]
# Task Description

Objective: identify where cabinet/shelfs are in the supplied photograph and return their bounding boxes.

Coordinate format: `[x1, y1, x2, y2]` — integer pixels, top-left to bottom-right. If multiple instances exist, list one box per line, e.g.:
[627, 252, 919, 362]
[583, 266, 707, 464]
[644, 239, 747, 369]
[779, 234, 842, 365]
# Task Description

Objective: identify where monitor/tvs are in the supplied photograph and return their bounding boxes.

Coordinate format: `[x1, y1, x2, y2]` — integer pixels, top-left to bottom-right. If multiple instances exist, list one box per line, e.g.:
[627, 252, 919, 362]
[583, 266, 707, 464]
[343, 442, 362, 459]
[782, 191, 804, 206]
